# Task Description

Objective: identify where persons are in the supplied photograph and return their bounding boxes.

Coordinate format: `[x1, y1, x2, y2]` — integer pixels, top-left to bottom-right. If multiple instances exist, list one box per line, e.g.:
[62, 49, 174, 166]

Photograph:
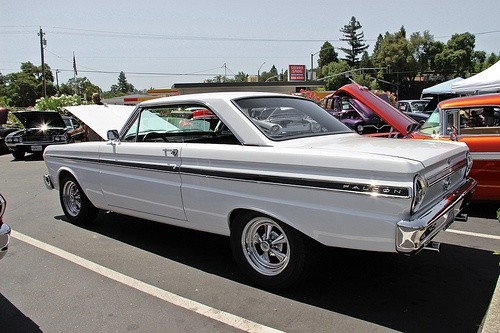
[413, 103, 420, 111]
[83, 93, 104, 141]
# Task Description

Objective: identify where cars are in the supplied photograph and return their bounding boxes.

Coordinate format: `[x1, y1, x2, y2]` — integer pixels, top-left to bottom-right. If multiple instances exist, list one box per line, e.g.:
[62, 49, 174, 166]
[403, 92, 500, 205]
[179, 83, 441, 136]
[0, 107, 87, 160]
[41, 92, 478, 292]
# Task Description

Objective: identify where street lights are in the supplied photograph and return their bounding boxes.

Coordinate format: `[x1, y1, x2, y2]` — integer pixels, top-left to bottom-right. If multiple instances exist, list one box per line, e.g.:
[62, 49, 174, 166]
[310, 46, 335, 81]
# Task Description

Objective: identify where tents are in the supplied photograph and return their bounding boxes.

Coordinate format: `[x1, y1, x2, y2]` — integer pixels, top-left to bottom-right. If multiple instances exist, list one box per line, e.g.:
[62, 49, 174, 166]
[452, 59, 500, 98]
[422, 77, 497, 102]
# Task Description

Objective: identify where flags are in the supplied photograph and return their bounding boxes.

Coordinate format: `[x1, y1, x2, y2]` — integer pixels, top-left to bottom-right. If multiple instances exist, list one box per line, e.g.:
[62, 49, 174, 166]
[73, 56, 78, 75]
